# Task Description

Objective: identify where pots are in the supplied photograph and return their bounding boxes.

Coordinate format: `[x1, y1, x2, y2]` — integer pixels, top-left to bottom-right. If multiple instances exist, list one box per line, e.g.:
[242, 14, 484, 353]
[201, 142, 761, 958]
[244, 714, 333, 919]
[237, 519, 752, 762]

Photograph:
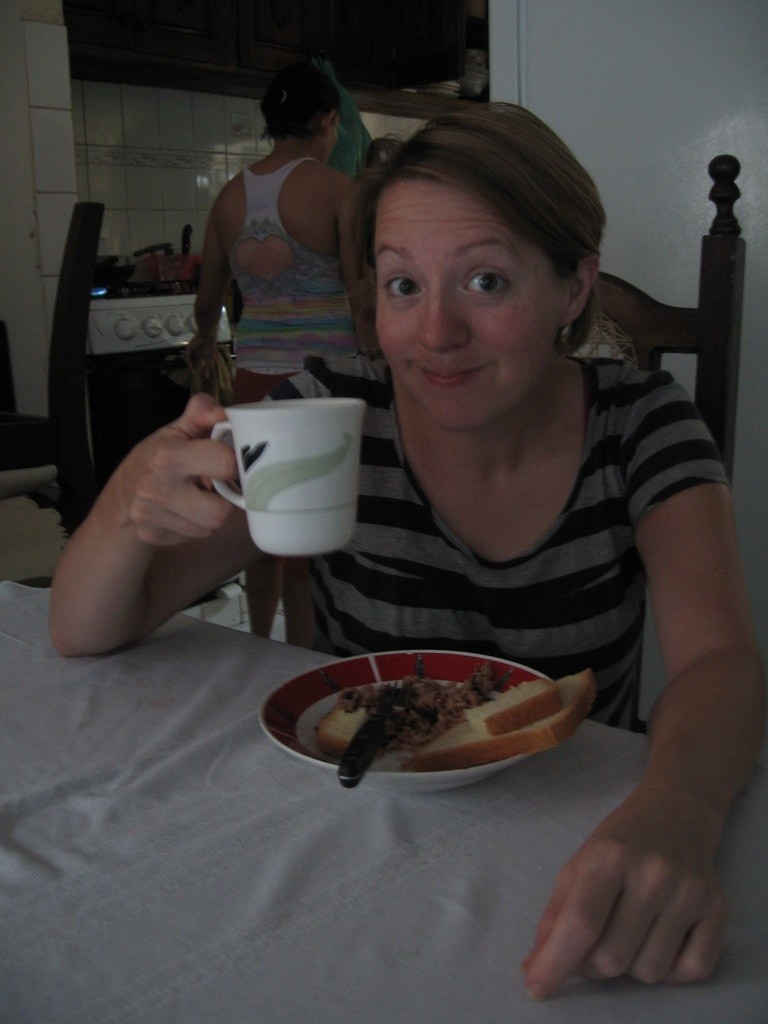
[94, 255, 136, 282]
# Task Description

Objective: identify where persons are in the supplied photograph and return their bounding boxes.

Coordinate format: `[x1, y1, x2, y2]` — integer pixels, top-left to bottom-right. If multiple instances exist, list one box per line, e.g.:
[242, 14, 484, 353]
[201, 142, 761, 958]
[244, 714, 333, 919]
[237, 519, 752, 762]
[183, 59, 387, 645]
[49, 101, 765, 1001]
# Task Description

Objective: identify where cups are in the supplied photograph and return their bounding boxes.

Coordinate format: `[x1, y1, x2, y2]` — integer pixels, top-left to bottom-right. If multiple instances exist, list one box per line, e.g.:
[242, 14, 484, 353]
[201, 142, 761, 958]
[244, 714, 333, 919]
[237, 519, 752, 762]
[212, 397, 365, 557]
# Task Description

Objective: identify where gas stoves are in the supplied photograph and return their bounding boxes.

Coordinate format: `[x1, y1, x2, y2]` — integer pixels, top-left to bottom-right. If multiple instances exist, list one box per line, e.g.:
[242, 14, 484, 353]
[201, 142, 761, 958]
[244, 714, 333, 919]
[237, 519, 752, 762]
[84, 278, 207, 300]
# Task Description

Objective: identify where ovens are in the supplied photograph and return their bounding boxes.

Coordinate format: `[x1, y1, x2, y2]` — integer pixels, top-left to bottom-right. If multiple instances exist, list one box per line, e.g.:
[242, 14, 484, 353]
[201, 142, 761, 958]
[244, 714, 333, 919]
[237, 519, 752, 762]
[87, 304, 231, 470]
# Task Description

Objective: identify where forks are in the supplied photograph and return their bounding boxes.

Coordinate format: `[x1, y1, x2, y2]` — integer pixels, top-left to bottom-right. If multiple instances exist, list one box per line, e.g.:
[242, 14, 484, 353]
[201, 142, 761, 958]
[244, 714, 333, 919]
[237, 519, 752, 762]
[336, 681, 410, 787]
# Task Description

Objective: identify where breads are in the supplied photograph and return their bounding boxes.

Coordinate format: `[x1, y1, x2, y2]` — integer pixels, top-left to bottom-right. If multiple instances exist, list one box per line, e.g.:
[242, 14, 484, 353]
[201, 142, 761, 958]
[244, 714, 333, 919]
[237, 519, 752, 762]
[461, 677, 563, 736]
[314, 701, 368, 758]
[414, 667, 595, 771]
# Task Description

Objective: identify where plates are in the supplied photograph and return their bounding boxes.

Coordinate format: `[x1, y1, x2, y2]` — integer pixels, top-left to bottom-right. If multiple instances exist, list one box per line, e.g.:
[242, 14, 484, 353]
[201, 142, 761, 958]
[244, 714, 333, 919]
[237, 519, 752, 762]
[418, 81, 461, 98]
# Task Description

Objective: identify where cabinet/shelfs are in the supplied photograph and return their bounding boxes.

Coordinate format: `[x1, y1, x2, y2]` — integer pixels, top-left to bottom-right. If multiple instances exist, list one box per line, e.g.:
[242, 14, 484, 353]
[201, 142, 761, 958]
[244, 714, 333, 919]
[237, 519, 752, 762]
[63, 0, 491, 124]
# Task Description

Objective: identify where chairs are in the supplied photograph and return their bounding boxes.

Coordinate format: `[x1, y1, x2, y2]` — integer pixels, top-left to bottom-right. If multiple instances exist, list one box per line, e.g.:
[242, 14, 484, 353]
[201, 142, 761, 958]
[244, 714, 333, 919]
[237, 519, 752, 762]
[585, 153, 748, 487]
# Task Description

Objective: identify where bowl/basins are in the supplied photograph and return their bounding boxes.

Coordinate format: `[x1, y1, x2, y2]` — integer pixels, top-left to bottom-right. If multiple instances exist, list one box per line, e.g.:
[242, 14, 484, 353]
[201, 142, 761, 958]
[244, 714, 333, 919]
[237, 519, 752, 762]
[256, 649, 553, 789]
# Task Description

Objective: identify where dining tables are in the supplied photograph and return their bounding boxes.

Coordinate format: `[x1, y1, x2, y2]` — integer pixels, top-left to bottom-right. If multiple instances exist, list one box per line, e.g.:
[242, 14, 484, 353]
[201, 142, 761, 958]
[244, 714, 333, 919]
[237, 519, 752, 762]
[0, 577, 767, 1024]
